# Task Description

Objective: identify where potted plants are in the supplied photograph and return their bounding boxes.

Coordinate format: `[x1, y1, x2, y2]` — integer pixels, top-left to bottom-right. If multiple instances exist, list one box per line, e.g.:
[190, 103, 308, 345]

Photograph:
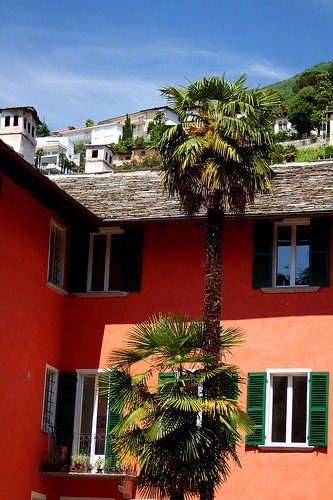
[48, 450, 131, 476]
